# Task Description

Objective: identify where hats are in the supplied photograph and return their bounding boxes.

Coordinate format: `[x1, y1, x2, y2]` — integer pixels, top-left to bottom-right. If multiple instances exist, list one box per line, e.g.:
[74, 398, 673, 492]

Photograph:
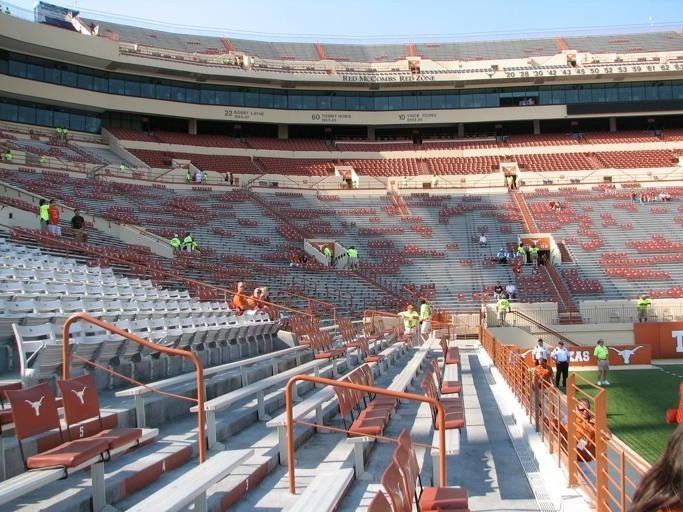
[407, 305, 414, 309]
[175, 234, 178, 238]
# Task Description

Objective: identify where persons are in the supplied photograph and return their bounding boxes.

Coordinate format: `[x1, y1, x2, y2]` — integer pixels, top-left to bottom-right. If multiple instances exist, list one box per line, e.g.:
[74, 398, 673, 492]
[630, 190, 671, 204]
[540, 252, 548, 266]
[258, 286, 276, 322]
[506, 250, 515, 263]
[346, 247, 356, 269]
[4, 150, 12, 160]
[352, 181, 358, 188]
[47, 198, 61, 237]
[554, 201, 560, 211]
[493, 280, 506, 303]
[289, 254, 316, 267]
[119, 162, 125, 173]
[530, 338, 549, 367]
[229, 172, 233, 185]
[183, 232, 192, 252]
[195, 170, 202, 183]
[36, 198, 57, 236]
[529, 244, 538, 264]
[4, 6, 10, 14]
[550, 341, 570, 388]
[229, 281, 250, 316]
[0, 150, 6, 160]
[38, 151, 47, 163]
[532, 357, 557, 416]
[191, 238, 200, 251]
[246, 287, 262, 311]
[88, 21, 95, 35]
[171, 233, 180, 251]
[70, 209, 86, 241]
[477, 232, 487, 246]
[516, 242, 525, 254]
[559, 399, 611, 487]
[496, 248, 506, 264]
[55, 125, 62, 139]
[222, 56, 256, 69]
[504, 280, 516, 303]
[495, 293, 511, 328]
[62, 126, 68, 139]
[506, 174, 513, 190]
[203, 172, 207, 183]
[396, 305, 418, 338]
[185, 172, 191, 183]
[192, 172, 196, 180]
[418, 299, 432, 342]
[603, 185, 616, 195]
[323, 244, 331, 257]
[627, 420, 682, 511]
[636, 294, 651, 324]
[593, 339, 611, 387]
[349, 246, 360, 268]
[224, 171, 228, 182]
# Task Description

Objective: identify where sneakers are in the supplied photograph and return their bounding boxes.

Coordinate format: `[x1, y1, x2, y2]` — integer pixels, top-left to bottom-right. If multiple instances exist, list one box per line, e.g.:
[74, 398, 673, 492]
[597, 380, 610, 385]
